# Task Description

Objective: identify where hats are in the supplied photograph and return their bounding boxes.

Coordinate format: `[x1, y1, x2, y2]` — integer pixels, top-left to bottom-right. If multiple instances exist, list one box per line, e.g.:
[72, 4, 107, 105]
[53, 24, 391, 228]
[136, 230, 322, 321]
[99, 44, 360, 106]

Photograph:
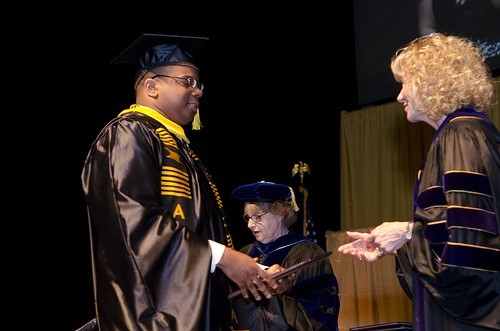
[110, 33, 209, 131]
[233, 181, 299, 212]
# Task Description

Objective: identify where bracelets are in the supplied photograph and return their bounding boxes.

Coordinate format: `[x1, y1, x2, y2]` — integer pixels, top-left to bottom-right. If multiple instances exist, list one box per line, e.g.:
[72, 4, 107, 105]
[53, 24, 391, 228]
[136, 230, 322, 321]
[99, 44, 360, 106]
[406, 222, 414, 241]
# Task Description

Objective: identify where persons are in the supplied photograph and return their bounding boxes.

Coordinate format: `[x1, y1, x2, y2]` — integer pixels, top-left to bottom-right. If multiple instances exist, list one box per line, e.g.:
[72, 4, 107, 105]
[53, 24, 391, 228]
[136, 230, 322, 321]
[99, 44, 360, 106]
[338, 34, 500, 331]
[81, 35, 295, 331]
[219, 181, 339, 331]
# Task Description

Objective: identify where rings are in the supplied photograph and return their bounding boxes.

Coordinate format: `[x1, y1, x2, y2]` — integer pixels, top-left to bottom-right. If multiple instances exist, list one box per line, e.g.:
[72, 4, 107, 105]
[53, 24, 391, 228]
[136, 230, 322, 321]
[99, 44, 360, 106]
[376, 248, 383, 256]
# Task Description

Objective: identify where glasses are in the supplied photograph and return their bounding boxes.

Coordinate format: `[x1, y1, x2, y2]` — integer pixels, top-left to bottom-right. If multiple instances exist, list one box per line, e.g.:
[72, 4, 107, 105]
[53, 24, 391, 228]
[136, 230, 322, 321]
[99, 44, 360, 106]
[243, 206, 275, 222]
[152, 74, 204, 91]
